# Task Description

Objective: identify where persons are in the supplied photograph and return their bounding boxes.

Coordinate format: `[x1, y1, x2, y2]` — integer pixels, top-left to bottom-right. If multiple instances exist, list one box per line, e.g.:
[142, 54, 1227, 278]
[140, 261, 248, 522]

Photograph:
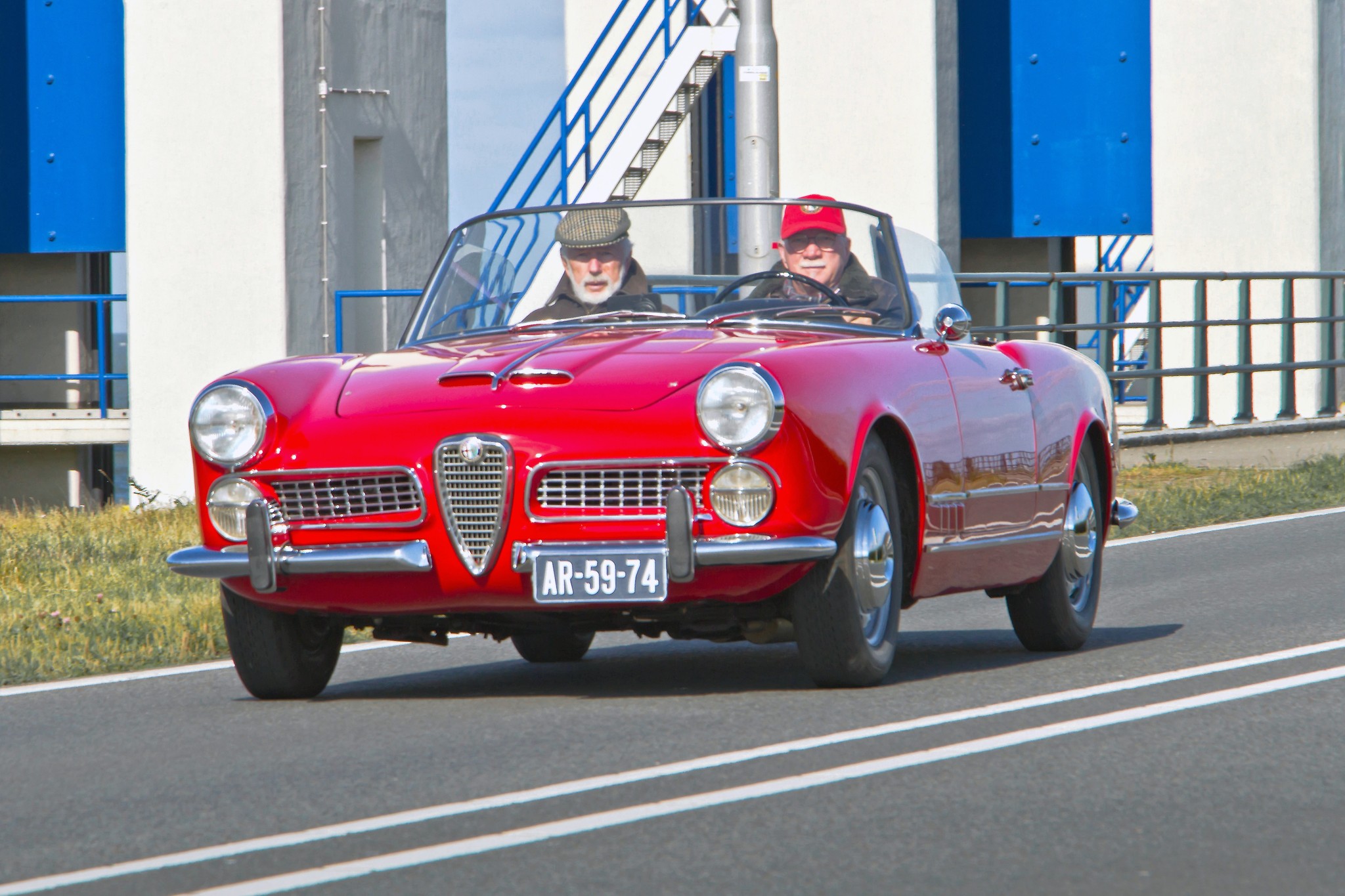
[734, 195, 920, 329]
[513, 205, 683, 323]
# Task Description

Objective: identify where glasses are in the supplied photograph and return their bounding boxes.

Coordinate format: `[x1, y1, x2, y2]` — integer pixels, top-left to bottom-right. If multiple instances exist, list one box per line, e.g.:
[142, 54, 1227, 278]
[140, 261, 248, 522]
[787, 234, 843, 253]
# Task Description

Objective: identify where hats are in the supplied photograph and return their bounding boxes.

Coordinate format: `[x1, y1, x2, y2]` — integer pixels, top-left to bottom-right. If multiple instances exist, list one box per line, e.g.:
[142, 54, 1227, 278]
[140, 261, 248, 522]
[782, 194, 846, 249]
[556, 208, 630, 247]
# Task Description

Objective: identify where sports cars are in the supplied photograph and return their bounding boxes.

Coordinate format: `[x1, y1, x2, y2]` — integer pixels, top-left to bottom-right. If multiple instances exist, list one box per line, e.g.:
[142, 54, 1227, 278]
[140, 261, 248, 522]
[166, 194, 1141, 700]
